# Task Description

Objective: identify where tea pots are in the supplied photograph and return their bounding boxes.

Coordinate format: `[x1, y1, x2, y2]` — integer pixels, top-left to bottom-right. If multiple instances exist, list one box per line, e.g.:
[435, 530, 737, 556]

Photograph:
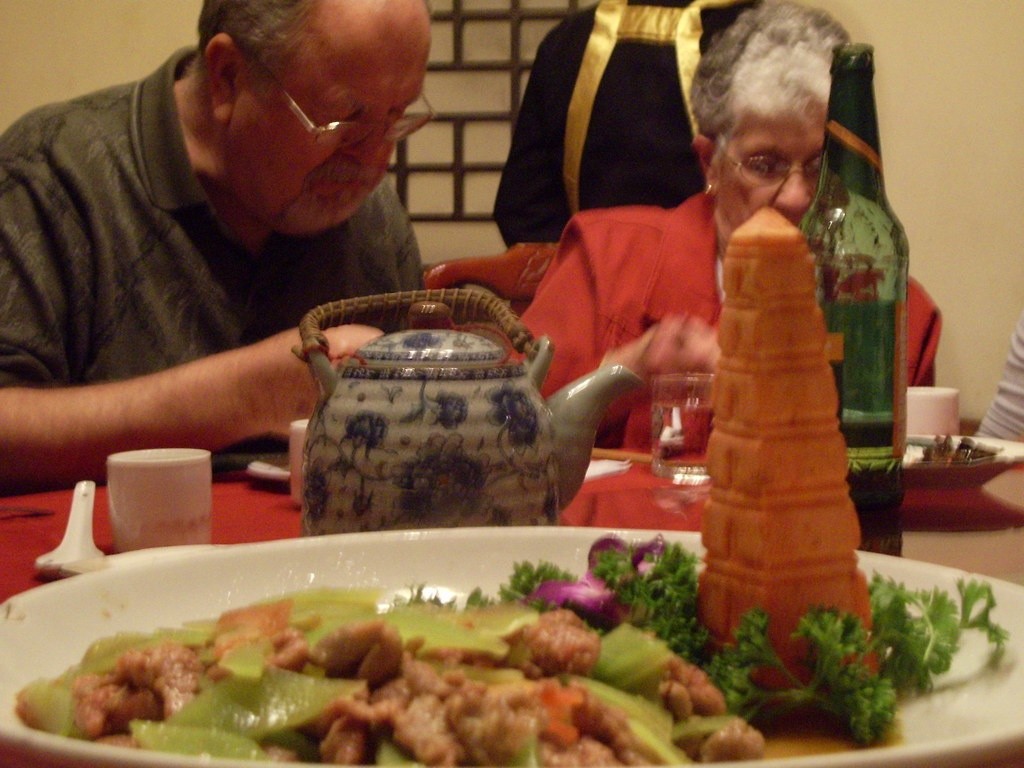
[288, 287, 640, 538]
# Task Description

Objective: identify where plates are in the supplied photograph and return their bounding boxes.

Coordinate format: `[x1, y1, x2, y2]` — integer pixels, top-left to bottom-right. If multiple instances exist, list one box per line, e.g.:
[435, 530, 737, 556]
[1, 528, 1024, 768]
[902, 433, 1024, 488]
[246, 456, 289, 481]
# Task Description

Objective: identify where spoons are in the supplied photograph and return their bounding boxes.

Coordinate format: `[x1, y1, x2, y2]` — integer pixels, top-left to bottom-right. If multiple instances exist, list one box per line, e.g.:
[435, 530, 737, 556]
[35, 479, 105, 579]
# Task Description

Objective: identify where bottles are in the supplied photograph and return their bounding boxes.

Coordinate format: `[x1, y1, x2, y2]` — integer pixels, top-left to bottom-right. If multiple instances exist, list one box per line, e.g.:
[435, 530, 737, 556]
[800, 43, 909, 511]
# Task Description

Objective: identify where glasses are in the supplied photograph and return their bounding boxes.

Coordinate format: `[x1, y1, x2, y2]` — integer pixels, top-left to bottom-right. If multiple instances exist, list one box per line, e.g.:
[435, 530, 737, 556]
[230, 35, 437, 147]
[714, 135, 825, 191]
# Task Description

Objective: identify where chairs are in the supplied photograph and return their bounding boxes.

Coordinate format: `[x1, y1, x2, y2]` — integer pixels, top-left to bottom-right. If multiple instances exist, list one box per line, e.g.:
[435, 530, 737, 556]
[424, 242, 560, 358]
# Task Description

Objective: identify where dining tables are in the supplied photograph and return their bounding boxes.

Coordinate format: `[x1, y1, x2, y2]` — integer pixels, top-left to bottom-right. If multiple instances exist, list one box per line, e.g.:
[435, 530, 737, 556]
[1, 447, 1023, 768]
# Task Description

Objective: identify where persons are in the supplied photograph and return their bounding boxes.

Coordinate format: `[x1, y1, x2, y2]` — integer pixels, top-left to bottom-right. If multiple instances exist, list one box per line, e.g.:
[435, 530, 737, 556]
[492, 0, 765, 250]
[974, 318, 1024, 441]
[514, 0, 941, 457]
[0, 0, 436, 482]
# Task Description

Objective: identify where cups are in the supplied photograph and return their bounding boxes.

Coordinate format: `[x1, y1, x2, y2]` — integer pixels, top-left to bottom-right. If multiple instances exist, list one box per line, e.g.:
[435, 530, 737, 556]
[649, 372, 714, 484]
[906, 386, 961, 436]
[106, 448, 211, 553]
[288, 419, 311, 508]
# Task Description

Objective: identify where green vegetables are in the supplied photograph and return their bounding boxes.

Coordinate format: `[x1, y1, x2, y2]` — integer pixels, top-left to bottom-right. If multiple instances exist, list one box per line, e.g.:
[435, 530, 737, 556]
[392, 540, 1010, 743]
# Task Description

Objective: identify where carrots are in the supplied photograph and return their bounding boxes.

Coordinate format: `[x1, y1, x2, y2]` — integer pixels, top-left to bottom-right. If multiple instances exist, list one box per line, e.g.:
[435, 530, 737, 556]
[693, 205, 881, 690]
